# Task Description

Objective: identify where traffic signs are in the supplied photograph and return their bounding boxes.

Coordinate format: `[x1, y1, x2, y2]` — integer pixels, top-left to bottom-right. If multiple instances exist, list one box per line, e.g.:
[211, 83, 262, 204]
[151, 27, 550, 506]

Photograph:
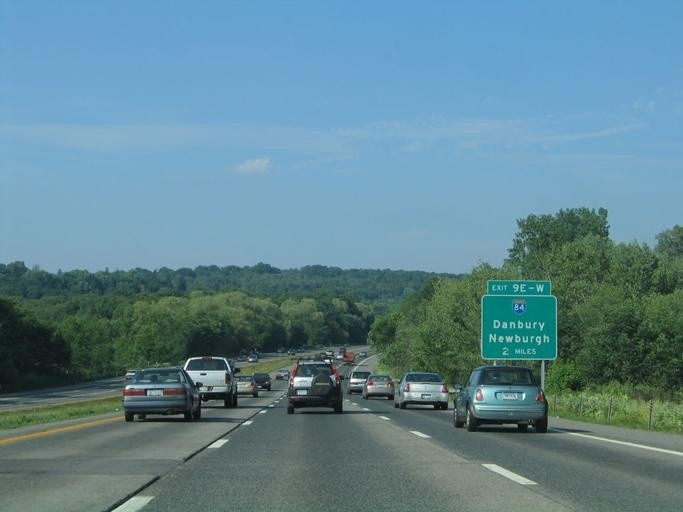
[479, 294, 558, 361]
[487, 280, 552, 297]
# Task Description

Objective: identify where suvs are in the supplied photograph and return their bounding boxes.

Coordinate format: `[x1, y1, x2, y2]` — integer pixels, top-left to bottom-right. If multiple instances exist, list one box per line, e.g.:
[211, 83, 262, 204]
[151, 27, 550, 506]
[251, 371, 271, 391]
[284, 355, 345, 415]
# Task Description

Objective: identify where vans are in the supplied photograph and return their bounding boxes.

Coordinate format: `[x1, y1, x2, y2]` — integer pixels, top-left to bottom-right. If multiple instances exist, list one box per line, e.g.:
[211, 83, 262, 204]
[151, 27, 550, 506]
[344, 368, 373, 397]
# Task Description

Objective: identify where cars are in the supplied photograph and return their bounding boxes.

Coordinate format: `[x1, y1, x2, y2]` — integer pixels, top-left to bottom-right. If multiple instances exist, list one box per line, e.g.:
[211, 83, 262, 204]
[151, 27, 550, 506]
[449, 363, 550, 435]
[235, 373, 259, 398]
[120, 367, 205, 423]
[123, 369, 137, 380]
[390, 372, 450, 411]
[362, 373, 393, 401]
[224, 342, 368, 389]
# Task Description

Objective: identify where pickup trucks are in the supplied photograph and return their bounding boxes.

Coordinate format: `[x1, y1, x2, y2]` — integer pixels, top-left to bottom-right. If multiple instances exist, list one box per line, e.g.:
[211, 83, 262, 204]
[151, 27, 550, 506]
[181, 355, 240, 409]
